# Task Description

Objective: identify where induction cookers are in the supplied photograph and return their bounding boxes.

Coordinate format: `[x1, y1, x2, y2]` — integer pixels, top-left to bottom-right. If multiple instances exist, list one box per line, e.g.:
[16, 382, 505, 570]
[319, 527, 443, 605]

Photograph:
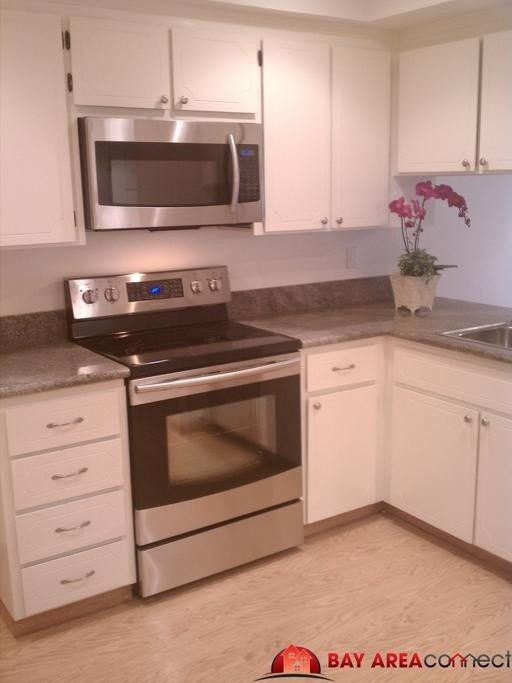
[63, 265, 303, 377]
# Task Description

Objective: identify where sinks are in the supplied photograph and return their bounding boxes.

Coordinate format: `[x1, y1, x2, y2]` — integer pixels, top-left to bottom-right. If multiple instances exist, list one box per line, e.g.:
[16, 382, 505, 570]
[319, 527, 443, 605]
[436, 321, 511, 352]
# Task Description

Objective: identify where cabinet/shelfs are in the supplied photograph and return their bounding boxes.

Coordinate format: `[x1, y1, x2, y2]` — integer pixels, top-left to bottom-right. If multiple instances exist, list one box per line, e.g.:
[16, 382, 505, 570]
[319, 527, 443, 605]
[261, 38, 393, 234]
[0, 17, 87, 252]
[299, 334, 385, 538]
[384, 336, 512, 581]
[63, 16, 263, 122]
[1, 378, 140, 637]
[396, 30, 512, 176]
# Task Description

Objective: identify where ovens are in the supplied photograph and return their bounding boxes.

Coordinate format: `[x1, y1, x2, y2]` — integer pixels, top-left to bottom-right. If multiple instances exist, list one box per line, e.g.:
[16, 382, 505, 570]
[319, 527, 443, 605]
[126, 349, 305, 599]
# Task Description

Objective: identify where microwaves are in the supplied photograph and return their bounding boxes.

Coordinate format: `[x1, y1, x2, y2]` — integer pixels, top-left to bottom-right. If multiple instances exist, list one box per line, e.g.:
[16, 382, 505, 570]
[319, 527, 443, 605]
[74, 116, 267, 232]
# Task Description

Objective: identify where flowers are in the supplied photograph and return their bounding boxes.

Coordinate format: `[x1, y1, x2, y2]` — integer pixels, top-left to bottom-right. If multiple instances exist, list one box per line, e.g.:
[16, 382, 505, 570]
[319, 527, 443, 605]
[388, 180, 471, 285]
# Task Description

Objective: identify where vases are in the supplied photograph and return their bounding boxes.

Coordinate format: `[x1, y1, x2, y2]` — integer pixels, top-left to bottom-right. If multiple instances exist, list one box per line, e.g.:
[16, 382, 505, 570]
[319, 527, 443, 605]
[389, 273, 442, 314]
